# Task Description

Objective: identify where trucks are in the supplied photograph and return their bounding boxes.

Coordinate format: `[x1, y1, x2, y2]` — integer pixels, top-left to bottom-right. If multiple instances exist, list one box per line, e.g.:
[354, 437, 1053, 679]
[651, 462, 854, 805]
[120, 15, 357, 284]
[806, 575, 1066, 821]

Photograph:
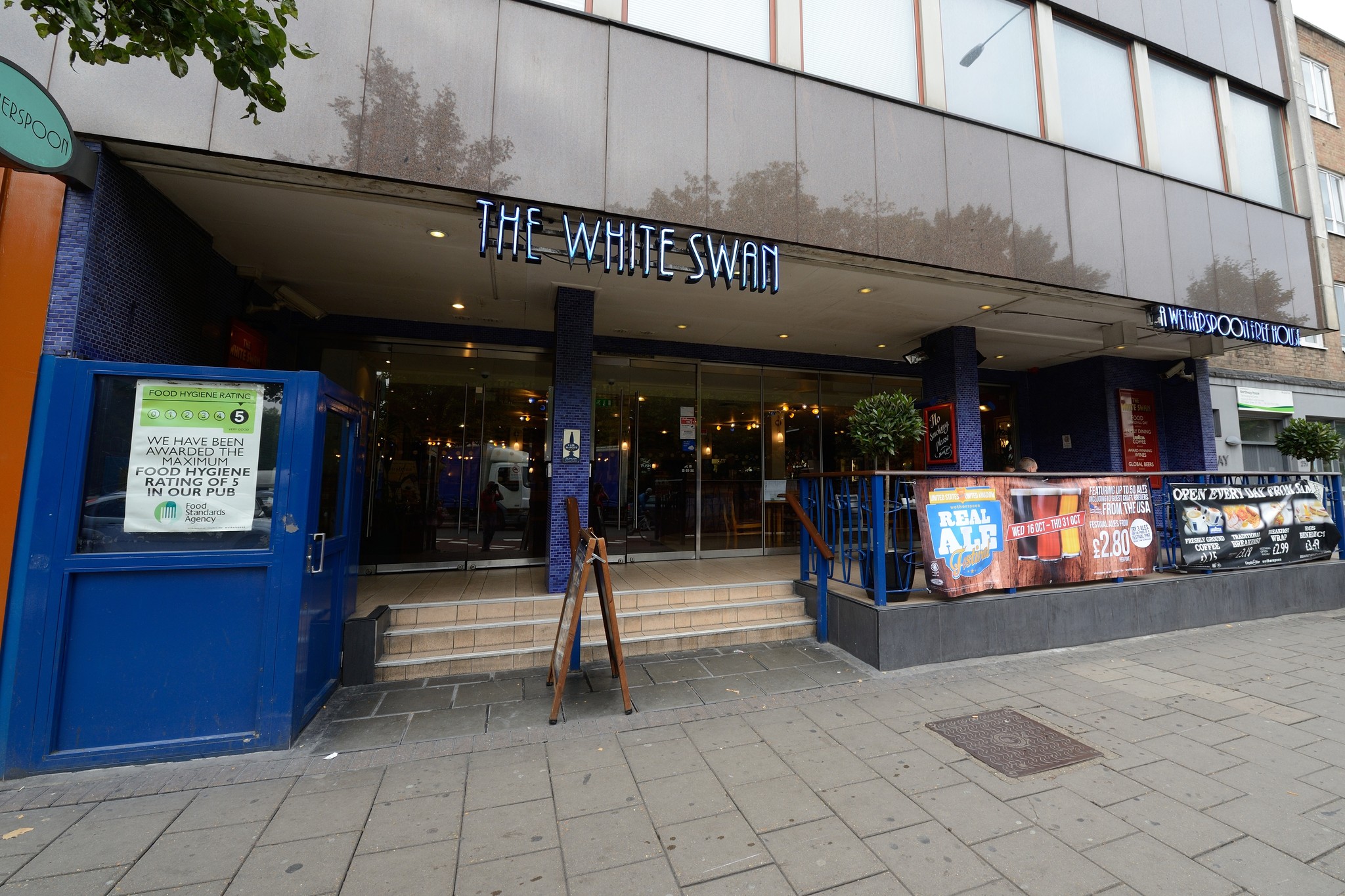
[426, 441, 531, 530]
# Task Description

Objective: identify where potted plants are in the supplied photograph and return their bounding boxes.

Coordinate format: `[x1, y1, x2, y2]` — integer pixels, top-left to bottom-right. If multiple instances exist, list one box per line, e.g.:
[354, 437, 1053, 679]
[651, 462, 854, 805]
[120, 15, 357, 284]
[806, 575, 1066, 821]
[845, 387, 930, 603]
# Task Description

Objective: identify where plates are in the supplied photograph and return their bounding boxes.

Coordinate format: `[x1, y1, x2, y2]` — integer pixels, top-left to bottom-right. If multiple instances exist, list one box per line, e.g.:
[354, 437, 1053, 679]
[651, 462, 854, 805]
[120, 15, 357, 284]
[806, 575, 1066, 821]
[1205, 515, 1224, 528]
[1225, 505, 1263, 531]
[1182, 515, 1188, 521]
[1182, 521, 1209, 536]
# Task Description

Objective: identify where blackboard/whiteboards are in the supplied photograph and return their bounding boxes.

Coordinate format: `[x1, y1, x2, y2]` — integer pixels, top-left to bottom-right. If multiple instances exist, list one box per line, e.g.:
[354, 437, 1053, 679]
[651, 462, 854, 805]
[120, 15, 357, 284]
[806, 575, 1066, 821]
[550, 526, 629, 694]
[924, 402, 958, 465]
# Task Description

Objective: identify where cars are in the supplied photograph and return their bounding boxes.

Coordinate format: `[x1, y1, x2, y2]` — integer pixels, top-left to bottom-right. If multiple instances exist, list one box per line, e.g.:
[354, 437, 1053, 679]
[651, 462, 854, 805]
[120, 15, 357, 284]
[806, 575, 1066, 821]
[628, 496, 656, 515]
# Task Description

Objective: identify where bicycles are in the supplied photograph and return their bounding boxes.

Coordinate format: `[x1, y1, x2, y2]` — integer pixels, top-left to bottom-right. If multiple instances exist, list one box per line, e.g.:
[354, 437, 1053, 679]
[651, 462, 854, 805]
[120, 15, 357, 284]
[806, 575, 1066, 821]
[626, 506, 656, 541]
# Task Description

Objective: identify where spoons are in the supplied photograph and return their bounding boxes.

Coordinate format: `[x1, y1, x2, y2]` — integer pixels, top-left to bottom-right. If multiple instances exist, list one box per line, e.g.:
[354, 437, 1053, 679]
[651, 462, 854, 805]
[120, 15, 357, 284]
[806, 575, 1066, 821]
[1184, 522, 1197, 534]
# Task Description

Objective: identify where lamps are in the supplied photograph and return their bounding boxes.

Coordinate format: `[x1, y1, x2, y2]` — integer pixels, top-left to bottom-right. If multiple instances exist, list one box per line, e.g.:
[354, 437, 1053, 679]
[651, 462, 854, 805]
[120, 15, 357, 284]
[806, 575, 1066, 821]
[730, 423, 735, 432]
[622, 426, 628, 450]
[716, 423, 724, 431]
[743, 424, 751, 430]
[638, 397, 645, 401]
[749, 423, 756, 428]
[782, 406, 792, 412]
[661, 430, 667, 434]
[777, 412, 783, 443]
[802, 404, 810, 410]
[651, 460, 656, 468]
[809, 409, 819, 414]
[704, 434, 710, 455]
[787, 407, 798, 418]
[1006, 421, 1012, 427]
[419, 396, 547, 474]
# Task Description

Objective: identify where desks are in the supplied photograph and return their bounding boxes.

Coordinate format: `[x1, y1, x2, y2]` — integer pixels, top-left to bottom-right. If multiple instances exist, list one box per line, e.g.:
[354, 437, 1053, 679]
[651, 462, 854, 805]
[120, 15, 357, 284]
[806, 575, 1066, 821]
[881, 501, 923, 550]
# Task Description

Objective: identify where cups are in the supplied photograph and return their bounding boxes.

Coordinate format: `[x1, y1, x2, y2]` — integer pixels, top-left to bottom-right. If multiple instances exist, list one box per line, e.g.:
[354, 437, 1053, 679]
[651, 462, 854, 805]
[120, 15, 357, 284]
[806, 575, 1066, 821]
[1057, 486, 1081, 559]
[1186, 510, 1206, 531]
[1029, 487, 1062, 562]
[1183, 506, 1197, 515]
[1201, 506, 1222, 524]
[1010, 488, 1037, 561]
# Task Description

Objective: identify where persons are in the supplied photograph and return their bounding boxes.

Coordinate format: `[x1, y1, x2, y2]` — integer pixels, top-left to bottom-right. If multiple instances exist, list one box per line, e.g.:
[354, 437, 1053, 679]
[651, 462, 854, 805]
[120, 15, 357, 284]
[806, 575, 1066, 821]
[1011, 456, 1046, 480]
[591, 482, 610, 540]
[1001, 465, 1016, 477]
[479, 482, 504, 552]
[630, 487, 652, 532]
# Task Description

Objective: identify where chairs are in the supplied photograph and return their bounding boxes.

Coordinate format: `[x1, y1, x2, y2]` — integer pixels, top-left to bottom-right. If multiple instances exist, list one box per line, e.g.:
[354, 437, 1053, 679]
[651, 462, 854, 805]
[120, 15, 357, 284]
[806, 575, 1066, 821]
[834, 494, 894, 563]
[721, 490, 762, 549]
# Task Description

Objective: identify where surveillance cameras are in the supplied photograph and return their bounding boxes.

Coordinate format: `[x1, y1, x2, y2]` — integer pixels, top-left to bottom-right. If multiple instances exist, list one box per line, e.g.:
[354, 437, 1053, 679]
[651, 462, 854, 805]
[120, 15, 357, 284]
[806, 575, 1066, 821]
[1158, 360, 1195, 382]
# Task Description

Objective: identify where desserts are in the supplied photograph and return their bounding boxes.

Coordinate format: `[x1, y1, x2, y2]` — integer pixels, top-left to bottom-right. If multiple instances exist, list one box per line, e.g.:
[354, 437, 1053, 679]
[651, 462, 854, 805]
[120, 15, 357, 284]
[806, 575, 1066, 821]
[1296, 501, 1330, 522]
